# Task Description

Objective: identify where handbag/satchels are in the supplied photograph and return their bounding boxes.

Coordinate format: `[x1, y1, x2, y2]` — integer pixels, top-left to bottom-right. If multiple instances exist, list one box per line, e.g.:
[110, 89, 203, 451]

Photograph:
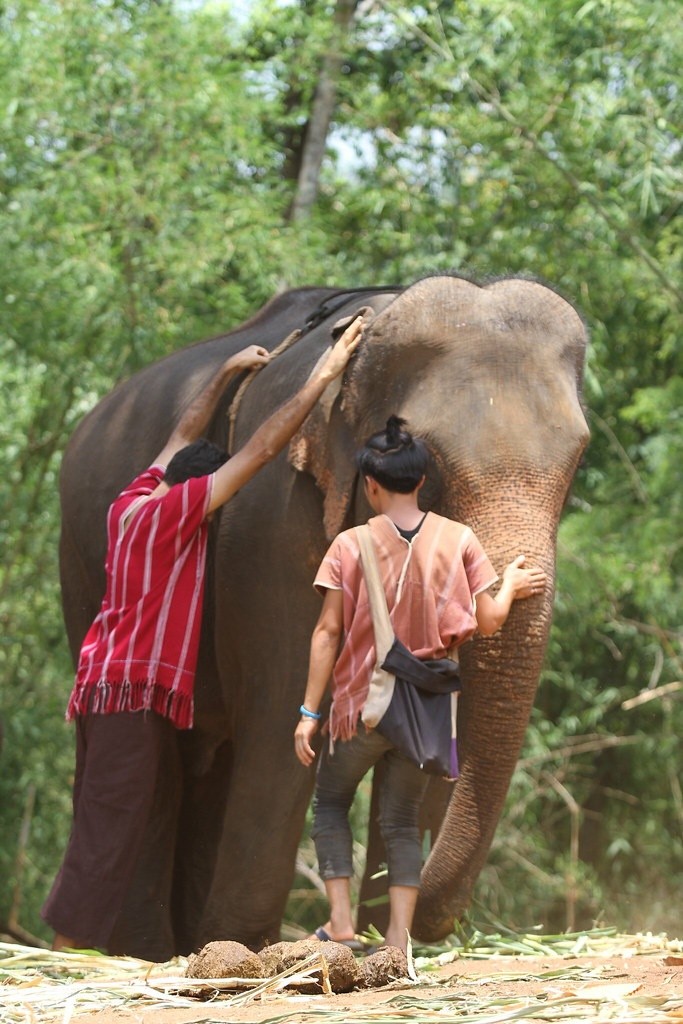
[358, 638, 465, 784]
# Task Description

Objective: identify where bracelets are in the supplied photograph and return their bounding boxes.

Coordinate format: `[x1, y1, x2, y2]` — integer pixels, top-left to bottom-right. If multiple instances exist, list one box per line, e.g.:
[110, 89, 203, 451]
[300, 705, 322, 719]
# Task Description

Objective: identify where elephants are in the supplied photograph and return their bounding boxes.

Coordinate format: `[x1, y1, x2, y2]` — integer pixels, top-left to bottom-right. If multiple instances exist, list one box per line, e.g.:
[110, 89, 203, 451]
[58, 272, 591, 950]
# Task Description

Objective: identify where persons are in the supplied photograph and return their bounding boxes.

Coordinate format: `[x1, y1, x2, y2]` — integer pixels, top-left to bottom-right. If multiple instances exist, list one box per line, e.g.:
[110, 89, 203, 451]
[295, 414, 547, 954]
[43, 315, 365, 962]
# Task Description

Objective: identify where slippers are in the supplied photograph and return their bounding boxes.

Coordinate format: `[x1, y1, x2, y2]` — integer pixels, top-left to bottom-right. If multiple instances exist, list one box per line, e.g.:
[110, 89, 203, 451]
[303, 928, 362, 950]
